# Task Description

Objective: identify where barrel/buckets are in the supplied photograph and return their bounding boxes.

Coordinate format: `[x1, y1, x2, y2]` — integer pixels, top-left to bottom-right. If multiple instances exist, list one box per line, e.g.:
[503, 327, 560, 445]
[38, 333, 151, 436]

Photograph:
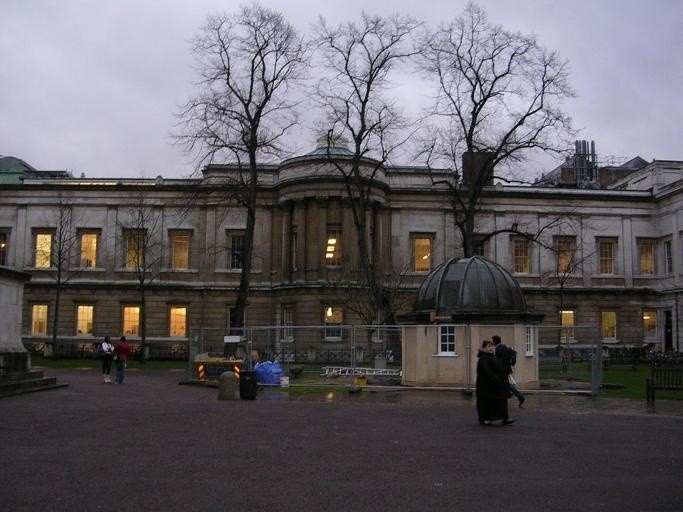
[279, 376, 291, 388]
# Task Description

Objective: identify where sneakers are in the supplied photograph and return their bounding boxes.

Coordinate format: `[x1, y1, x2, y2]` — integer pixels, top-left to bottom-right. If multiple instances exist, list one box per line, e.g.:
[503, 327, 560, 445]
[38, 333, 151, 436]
[479, 419, 515, 425]
[517, 397, 525, 406]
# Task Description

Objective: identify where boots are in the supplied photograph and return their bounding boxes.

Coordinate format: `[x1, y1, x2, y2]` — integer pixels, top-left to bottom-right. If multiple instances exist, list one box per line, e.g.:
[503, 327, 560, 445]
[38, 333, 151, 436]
[102, 374, 112, 384]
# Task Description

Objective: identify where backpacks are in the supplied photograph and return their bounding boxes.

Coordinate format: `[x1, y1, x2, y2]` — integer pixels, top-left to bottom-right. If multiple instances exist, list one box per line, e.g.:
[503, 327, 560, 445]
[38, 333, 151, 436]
[496, 343, 515, 365]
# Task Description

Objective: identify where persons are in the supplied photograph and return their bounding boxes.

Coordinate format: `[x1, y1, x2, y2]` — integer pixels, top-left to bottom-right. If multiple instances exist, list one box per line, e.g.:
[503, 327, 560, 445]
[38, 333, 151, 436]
[111, 335, 130, 385]
[491, 335, 525, 406]
[475, 339, 515, 425]
[100, 336, 115, 383]
